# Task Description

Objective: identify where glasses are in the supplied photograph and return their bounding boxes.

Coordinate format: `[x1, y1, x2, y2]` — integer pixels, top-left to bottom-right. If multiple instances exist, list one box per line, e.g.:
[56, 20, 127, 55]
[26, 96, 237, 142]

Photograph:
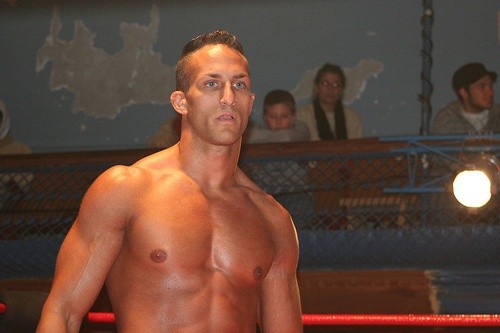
[318, 81, 342, 88]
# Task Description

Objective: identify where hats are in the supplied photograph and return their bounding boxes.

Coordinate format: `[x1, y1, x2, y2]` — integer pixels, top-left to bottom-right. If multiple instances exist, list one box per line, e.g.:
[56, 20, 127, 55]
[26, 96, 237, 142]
[452, 63, 498, 87]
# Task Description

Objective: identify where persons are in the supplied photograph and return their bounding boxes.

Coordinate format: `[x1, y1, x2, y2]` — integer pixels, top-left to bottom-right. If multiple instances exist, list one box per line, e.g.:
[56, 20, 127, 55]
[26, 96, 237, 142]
[243, 89, 311, 144]
[35, 28, 304, 333]
[146, 115, 182, 149]
[294, 62, 363, 143]
[0, 100, 33, 156]
[431, 63, 500, 135]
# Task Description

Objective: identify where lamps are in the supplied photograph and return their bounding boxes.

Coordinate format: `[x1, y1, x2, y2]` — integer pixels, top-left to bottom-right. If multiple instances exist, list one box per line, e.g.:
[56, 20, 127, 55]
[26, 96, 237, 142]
[452, 154, 500, 207]
